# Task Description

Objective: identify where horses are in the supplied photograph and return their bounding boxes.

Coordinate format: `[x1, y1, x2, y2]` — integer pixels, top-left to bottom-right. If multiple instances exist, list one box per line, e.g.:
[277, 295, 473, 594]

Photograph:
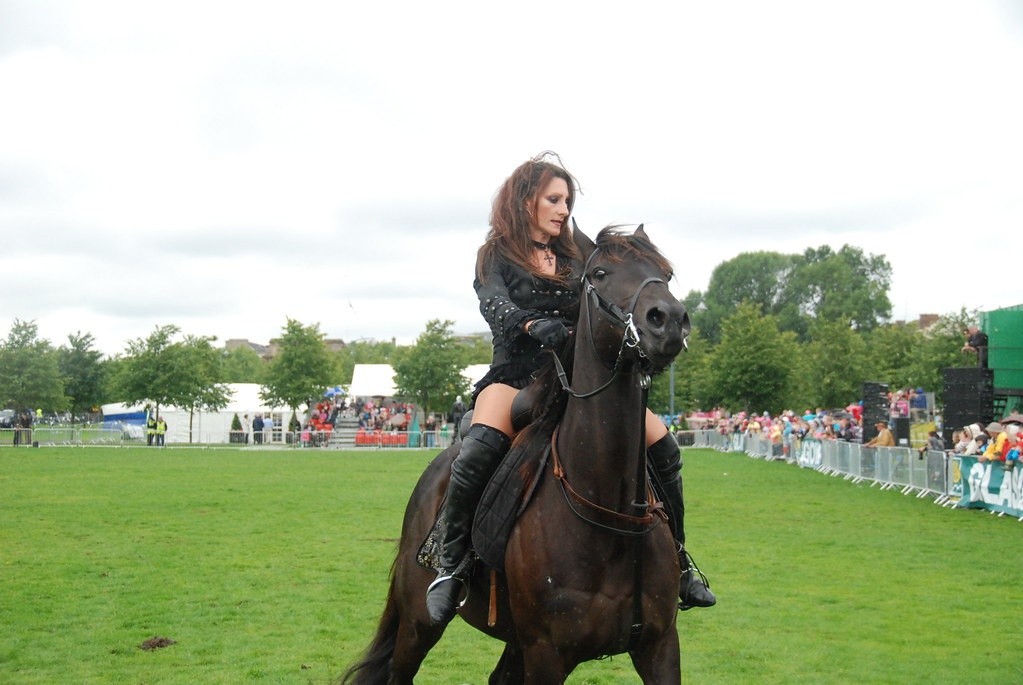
[335, 216, 691, 685]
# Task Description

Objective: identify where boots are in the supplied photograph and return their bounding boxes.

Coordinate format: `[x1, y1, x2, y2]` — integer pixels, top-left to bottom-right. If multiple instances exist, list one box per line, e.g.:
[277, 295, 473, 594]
[648, 430, 716, 609]
[425, 421, 511, 625]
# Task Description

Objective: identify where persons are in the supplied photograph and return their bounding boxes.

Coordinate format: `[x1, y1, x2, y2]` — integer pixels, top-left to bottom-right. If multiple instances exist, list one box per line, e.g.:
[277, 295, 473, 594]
[961, 324, 988, 367]
[655, 386, 1023, 469]
[10, 410, 33, 447]
[426, 157, 718, 624]
[294, 391, 467, 448]
[145, 415, 168, 445]
[242, 412, 274, 444]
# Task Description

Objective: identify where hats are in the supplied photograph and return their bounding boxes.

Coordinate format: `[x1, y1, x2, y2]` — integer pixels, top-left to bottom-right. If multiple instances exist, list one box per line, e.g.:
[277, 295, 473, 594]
[985, 422, 1002, 433]
